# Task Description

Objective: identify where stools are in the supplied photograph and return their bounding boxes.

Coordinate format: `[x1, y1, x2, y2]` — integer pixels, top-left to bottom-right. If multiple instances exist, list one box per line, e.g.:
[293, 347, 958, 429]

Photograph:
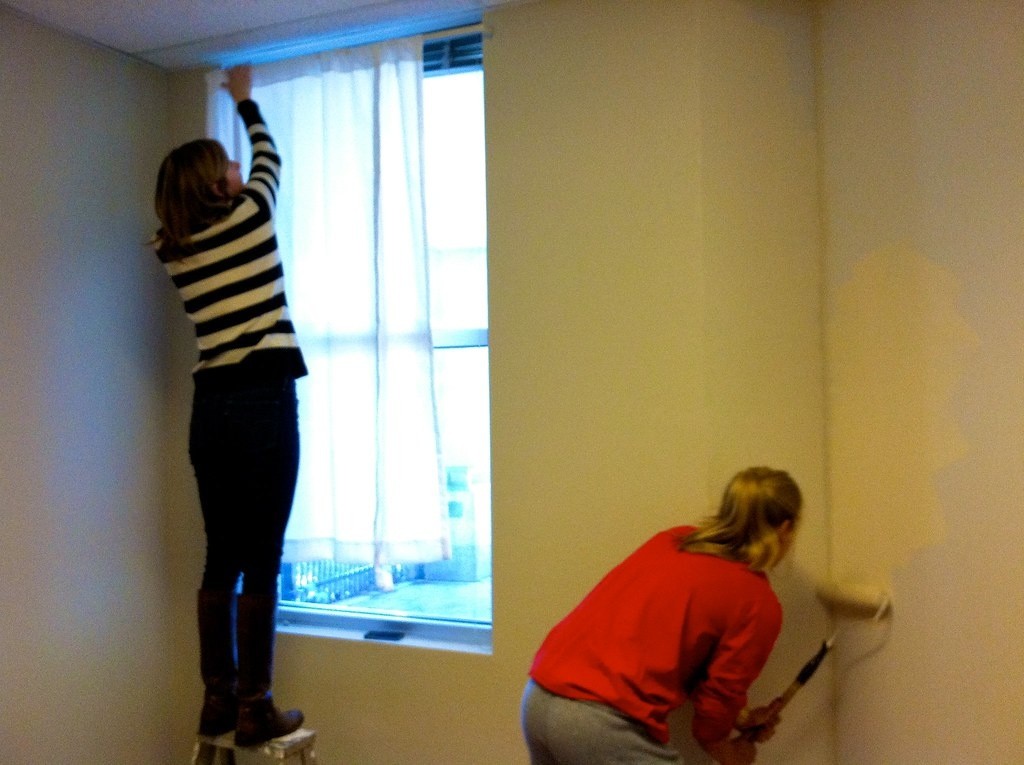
[191, 729, 317, 765]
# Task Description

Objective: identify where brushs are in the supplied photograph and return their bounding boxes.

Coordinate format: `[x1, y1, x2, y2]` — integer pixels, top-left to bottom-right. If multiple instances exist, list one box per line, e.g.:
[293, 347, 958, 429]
[737, 580, 892, 741]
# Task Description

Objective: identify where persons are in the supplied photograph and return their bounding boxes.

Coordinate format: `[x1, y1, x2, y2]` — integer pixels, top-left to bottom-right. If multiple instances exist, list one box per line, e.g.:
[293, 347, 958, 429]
[154, 61, 309, 748]
[521, 466, 804, 765]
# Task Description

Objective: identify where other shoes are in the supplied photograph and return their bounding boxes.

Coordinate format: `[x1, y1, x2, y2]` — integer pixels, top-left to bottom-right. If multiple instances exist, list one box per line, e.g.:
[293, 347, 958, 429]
[234, 696, 304, 747]
[199, 690, 240, 736]
